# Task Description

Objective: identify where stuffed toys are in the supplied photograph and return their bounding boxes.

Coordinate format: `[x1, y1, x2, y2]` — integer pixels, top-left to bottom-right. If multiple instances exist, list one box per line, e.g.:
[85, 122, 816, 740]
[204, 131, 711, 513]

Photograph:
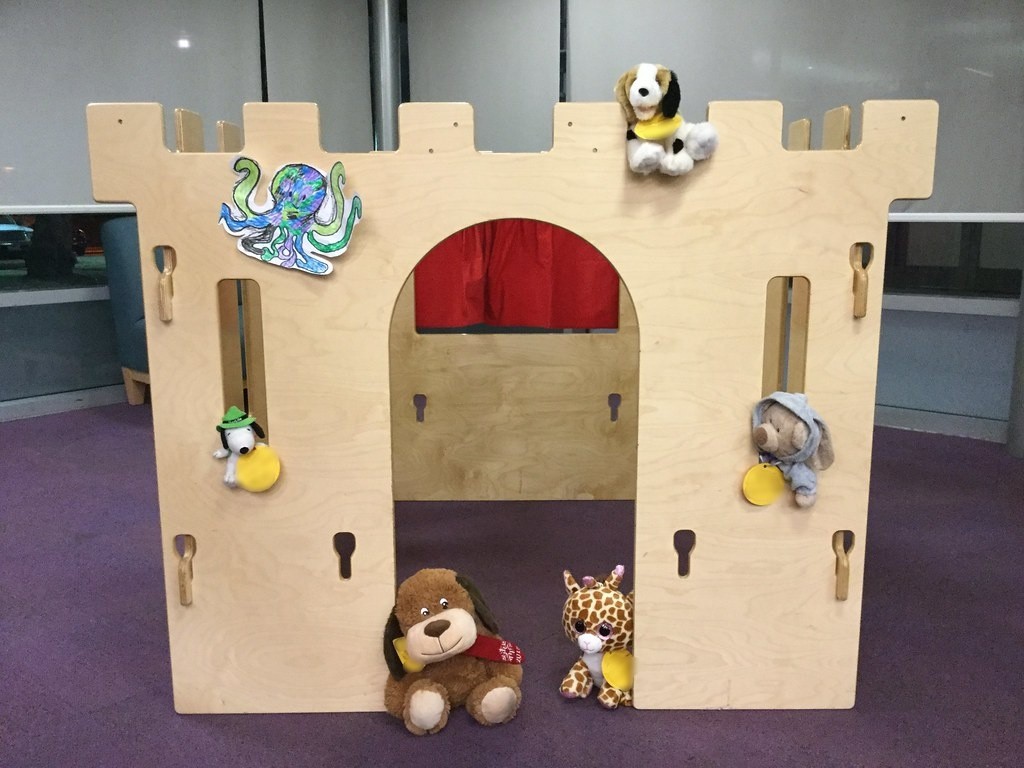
[741, 388, 833, 513]
[213, 406, 278, 494]
[615, 59, 717, 182]
[379, 564, 527, 734]
[555, 565, 635, 710]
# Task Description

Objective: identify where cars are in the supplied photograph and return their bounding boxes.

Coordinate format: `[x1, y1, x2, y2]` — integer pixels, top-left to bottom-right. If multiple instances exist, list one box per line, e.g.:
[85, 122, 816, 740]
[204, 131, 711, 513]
[67, 226, 88, 255]
[0, 213, 34, 257]
